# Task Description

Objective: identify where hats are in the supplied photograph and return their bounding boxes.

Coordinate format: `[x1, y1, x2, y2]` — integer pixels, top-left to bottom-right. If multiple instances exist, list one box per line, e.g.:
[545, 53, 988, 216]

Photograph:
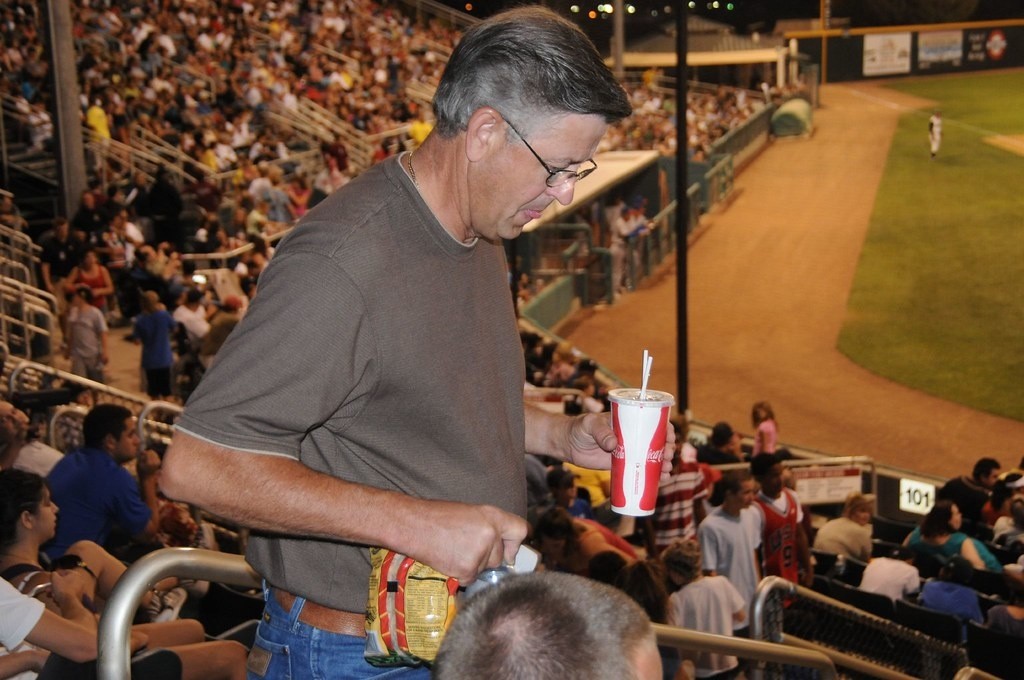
[547, 469, 580, 487]
[660, 540, 702, 568]
[76, 286, 93, 303]
[846, 492, 876, 508]
[934, 554, 972, 583]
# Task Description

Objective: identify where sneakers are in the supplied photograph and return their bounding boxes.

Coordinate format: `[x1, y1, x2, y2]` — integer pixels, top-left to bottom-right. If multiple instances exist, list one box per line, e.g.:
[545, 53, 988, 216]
[180, 522, 214, 594]
[150, 588, 186, 622]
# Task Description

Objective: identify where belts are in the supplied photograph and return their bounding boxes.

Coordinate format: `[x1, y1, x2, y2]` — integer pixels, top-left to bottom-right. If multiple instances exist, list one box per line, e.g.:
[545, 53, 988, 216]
[270, 587, 368, 637]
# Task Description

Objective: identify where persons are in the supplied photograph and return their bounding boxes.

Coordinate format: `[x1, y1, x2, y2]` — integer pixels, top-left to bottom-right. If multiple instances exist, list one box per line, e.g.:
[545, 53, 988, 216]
[928, 111, 944, 159]
[0, 246, 1024, 680]
[158, 9, 677, 680]
[0, 0, 822, 317]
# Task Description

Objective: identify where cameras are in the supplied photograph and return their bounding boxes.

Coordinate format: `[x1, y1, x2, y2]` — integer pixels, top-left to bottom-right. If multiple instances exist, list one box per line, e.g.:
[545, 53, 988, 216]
[463, 544, 542, 601]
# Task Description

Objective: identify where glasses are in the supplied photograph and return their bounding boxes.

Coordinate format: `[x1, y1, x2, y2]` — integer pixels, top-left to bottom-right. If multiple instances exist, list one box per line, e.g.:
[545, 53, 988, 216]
[505, 120, 597, 187]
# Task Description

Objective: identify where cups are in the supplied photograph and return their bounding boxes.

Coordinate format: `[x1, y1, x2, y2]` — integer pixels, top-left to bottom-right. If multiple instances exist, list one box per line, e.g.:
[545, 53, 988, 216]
[606, 386, 677, 519]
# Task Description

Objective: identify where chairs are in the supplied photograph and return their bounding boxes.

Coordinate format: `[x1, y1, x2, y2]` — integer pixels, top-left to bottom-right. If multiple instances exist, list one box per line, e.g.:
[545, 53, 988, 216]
[798, 517, 1024, 680]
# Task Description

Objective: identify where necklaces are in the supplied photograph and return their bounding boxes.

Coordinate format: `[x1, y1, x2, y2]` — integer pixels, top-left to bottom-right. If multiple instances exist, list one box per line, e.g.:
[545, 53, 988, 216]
[407, 151, 419, 189]
